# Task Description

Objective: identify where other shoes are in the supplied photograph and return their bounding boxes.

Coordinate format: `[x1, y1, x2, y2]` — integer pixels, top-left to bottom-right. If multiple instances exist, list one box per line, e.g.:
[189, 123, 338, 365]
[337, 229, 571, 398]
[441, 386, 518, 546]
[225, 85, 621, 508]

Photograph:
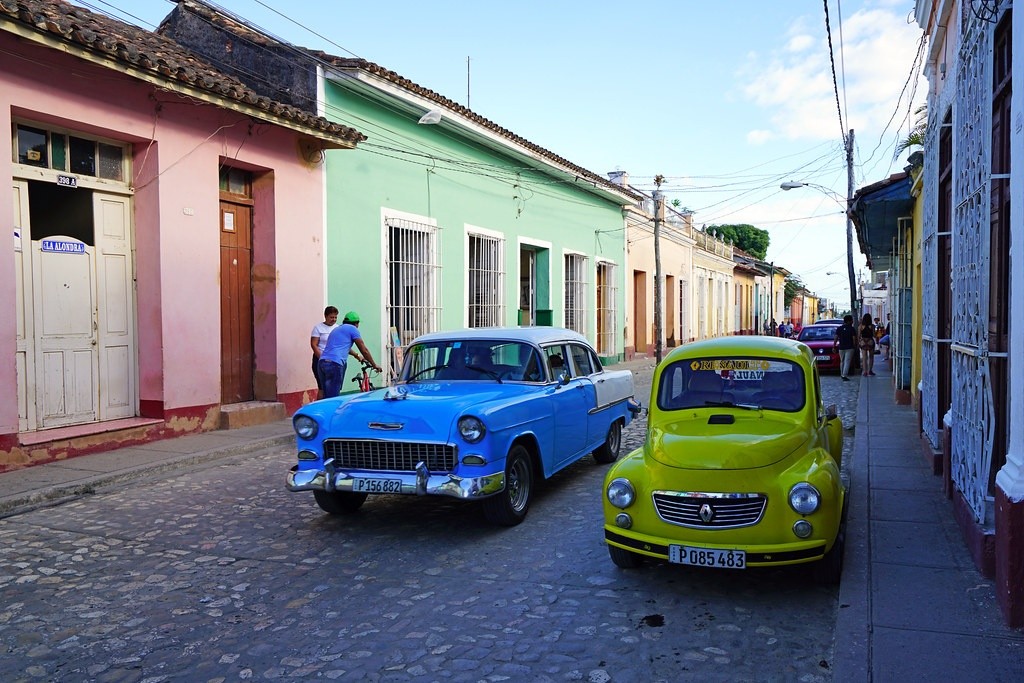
[863, 372, 869, 376]
[880, 356, 888, 362]
[869, 370, 876, 375]
[880, 346, 882, 348]
[843, 377, 850, 383]
[841, 377, 844, 379]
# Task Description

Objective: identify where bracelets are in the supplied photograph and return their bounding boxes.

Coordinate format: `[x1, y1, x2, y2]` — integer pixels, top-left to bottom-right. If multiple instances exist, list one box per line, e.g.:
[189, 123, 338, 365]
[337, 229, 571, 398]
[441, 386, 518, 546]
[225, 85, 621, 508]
[354, 353, 360, 359]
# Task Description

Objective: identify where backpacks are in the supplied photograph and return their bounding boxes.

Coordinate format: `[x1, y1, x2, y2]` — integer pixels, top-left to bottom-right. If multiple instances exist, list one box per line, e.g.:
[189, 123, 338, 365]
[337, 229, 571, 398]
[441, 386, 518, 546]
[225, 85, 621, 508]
[861, 324, 873, 339]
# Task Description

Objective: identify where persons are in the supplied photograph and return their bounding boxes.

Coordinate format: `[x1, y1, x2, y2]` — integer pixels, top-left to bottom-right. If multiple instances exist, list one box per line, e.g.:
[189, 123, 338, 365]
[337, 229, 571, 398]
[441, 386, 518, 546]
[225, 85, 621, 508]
[311, 306, 366, 401]
[763, 318, 802, 340]
[433, 346, 479, 380]
[470, 346, 495, 371]
[832, 315, 857, 381]
[872, 313, 893, 363]
[317, 311, 383, 399]
[546, 352, 565, 381]
[508, 343, 546, 383]
[857, 312, 878, 378]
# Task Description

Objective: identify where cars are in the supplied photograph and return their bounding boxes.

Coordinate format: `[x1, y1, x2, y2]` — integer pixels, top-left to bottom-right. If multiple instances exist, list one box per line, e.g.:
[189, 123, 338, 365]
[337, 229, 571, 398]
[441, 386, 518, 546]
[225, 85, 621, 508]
[791, 323, 853, 373]
[285, 325, 643, 526]
[604, 336, 845, 576]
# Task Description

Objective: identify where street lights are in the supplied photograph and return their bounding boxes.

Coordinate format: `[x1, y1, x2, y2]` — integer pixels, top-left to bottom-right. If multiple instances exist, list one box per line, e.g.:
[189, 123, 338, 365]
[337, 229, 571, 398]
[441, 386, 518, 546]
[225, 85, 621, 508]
[779, 182, 860, 368]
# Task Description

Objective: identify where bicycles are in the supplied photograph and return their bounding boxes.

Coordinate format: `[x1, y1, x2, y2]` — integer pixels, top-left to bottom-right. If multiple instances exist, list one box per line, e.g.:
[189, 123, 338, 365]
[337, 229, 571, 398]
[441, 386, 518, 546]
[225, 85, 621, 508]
[352, 359, 384, 393]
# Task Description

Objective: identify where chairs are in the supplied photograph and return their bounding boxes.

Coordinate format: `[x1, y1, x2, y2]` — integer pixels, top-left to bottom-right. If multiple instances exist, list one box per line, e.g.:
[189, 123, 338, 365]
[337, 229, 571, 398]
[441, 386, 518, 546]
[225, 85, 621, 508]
[747, 371, 803, 408]
[680, 370, 737, 408]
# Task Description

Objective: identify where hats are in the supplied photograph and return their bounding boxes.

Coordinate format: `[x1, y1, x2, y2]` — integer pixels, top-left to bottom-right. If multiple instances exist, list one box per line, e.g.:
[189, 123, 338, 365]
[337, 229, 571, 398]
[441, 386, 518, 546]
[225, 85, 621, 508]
[343, 311, 360, 322]
